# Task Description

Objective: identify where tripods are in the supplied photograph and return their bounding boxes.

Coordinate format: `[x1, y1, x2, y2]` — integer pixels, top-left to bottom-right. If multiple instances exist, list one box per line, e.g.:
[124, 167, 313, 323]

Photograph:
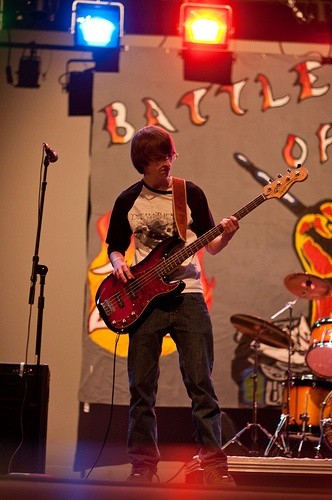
[216, 317, 332, 459]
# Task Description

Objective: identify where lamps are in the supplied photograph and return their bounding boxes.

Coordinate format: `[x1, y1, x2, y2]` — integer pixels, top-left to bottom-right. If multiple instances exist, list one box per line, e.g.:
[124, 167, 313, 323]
[14, 46, 44, 88]
[71, 0, 124, 73]
[63, 59, 94, 116]
[178, 2, 235, 86]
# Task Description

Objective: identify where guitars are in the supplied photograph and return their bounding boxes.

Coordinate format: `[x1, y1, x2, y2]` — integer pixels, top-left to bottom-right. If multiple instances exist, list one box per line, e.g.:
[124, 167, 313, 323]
[95, 164, 308, 334]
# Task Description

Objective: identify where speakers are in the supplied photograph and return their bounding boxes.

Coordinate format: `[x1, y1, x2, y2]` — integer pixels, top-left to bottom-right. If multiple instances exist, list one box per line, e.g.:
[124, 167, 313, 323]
[0, 362, 49, 474]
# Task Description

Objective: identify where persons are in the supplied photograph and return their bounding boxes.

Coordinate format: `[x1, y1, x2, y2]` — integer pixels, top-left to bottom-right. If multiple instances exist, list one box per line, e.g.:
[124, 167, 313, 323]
[106, 125, 239, 486]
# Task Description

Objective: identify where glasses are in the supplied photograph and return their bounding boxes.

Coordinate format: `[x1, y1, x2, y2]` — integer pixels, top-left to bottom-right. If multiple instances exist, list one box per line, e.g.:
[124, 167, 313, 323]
[147, 153, 178, 165]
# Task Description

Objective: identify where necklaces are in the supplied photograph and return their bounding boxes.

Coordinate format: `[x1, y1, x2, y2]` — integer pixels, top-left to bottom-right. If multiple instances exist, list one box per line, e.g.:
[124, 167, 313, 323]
[142, 176, 170, 200]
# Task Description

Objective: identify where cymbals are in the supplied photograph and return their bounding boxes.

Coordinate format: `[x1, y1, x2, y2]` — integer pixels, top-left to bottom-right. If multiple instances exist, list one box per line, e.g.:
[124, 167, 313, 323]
[230, 314, 294, 348]
[283, 272, 328, 299]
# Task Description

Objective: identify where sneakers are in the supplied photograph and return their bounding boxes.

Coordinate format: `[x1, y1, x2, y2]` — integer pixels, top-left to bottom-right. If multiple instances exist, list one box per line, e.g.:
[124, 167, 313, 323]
[204, 465, 236, 485]
[125, 466, 154, 483]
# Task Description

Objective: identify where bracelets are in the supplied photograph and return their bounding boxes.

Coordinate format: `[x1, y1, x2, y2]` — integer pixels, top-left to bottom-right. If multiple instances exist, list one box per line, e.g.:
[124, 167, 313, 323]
[112, 257, 121, 263]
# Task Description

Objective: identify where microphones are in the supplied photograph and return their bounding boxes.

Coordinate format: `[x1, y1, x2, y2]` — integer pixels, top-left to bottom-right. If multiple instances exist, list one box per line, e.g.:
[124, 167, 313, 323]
[43, 142, 58, 163]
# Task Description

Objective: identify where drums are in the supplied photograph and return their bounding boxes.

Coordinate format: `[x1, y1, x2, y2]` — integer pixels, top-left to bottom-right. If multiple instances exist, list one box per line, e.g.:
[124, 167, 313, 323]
[281, 379, 332, 428]
[320, 391, 332, 451]
[305, 319, 332, 380]
